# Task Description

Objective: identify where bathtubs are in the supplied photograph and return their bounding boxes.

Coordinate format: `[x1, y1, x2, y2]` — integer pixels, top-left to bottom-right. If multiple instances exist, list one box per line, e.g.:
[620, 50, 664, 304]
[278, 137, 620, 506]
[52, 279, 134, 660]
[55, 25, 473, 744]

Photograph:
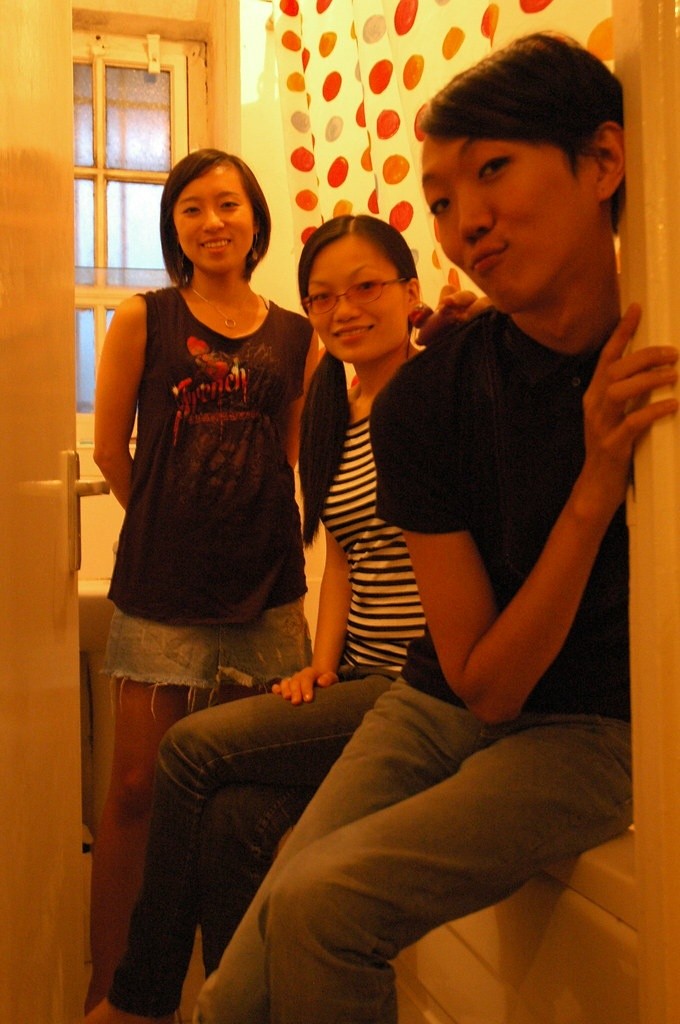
[384, 828, 637, 1024]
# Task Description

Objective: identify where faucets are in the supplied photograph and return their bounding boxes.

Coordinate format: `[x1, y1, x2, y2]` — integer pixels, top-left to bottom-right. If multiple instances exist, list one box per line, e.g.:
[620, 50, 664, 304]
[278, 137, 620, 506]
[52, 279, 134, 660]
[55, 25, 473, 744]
[112, 540, 119, 565]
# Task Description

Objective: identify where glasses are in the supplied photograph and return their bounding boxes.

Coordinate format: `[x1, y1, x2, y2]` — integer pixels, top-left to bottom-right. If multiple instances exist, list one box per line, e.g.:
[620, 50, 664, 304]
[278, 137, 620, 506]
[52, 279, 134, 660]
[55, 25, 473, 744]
[301, 276, 406, 315]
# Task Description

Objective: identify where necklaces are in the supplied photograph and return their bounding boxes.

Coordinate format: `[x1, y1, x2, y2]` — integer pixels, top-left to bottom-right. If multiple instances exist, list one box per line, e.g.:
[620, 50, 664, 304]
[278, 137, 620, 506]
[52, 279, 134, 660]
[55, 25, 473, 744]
[192, 288, 251, 329]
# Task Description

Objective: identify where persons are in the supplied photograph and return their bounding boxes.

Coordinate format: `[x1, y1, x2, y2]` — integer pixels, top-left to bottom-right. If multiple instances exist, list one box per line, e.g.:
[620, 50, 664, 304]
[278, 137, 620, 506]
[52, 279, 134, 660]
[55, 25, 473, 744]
[86, 148, 320, 1012]
[190, 30, 680, 1024]
[86, 216, 498, 1023]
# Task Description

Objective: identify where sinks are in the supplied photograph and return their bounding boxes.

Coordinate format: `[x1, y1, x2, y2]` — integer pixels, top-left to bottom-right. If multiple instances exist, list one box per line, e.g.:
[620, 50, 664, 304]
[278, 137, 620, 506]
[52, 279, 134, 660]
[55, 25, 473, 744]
[78, 579, 116, 651]
